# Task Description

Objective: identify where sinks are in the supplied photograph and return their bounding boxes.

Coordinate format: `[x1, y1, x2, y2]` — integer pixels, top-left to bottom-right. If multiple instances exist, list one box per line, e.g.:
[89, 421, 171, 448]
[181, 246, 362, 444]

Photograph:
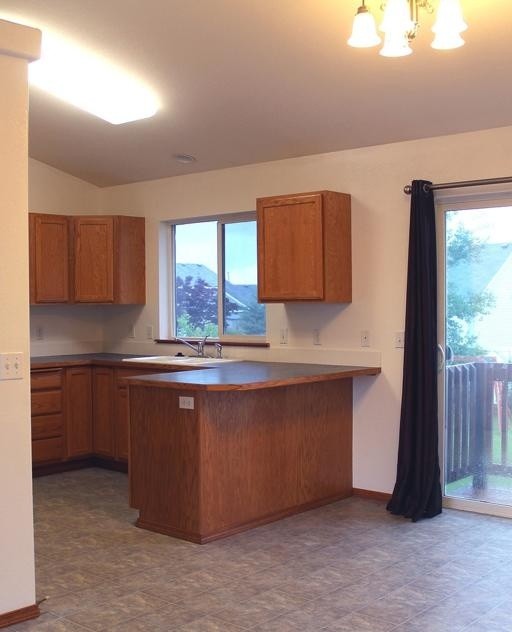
[121, 356, 238, 366]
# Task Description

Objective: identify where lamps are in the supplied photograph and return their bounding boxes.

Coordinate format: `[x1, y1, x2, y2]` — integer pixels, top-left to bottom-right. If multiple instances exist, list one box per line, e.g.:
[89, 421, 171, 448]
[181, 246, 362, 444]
[347, 0, 469, 58]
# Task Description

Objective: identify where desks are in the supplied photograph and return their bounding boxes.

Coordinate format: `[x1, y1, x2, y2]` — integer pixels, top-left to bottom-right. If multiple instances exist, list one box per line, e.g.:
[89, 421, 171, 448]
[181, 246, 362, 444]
[121, 354, 382, 545]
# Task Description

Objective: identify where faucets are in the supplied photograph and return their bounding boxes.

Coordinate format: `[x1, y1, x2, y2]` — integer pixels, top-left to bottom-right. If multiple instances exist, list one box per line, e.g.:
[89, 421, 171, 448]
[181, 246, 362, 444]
[175, 336, 208, 356]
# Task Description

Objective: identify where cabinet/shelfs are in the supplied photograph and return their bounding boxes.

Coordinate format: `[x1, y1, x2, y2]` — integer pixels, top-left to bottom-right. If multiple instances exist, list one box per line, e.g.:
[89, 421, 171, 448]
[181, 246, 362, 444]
[30, 361, 69, 471]
[254, 188, 353, 305]
[91, 360, 115, 462]
[65, 360, 94, 461]
[70, 213, 147, 306]
[116, 363, 169, 468]
[28, 212, 76, 306]
